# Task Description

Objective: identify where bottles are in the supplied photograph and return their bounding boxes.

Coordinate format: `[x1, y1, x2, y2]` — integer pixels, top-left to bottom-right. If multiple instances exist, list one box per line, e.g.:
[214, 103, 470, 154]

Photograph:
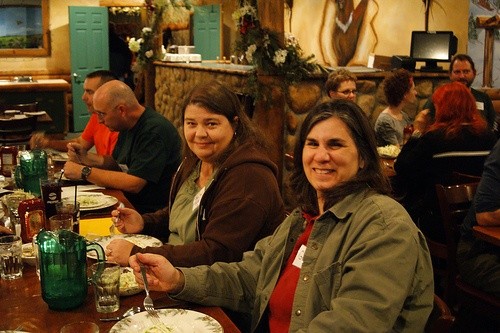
[18, 198, 47, 243]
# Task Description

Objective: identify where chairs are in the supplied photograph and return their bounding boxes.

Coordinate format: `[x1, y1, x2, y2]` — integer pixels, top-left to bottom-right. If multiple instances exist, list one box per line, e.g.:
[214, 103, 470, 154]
[425, 170, 481, 333]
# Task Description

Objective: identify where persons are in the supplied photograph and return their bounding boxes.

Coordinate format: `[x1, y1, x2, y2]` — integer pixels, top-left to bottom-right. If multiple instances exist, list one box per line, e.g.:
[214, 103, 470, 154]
[105, 80, 286, 267]
[395, 82, 497, 245]
[63, 80, 183, 212]
[423, 54, 494, 131]
[129, 98, 435, 333]
[326, 68, 357, 103]
[456, 138, 500, 300]
[29, 70, 121, 156]
[375, 69, 430, 144]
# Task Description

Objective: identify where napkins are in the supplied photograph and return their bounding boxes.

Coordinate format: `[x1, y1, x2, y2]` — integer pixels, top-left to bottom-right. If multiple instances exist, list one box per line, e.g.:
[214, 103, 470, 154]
[61, 185, 106, 192]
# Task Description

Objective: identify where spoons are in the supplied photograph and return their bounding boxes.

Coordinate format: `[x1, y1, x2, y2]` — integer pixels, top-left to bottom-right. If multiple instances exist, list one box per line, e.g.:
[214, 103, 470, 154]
[120, 302, 183, 317]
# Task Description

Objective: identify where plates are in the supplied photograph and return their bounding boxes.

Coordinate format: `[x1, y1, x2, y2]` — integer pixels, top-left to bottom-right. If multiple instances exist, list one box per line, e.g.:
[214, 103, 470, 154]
[0, 175, 39, 234]
[376, 147, 401, 158]
[87, 234, 163, 261]
[54, 170, 86, 180]
[109, 309, 224, 333]
[50, 151, 70, 162]
[62, 194, 119, 211]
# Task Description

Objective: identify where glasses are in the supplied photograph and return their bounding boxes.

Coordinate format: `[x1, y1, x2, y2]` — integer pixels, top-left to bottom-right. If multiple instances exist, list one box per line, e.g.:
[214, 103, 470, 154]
[333, 88, 358, 98]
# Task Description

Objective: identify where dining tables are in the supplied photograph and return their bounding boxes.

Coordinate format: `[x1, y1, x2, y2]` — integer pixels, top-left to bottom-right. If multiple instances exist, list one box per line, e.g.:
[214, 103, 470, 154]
[0, 160, 240, 333]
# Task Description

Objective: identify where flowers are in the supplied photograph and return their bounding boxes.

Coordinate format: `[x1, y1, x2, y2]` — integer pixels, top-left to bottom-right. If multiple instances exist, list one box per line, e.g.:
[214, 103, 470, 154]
[126, 27, 154, 65]
[233, 0, 318, 107]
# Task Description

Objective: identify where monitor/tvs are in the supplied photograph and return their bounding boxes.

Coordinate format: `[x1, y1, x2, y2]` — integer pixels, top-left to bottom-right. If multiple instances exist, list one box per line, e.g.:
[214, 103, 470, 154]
[410, 30, 451, 73]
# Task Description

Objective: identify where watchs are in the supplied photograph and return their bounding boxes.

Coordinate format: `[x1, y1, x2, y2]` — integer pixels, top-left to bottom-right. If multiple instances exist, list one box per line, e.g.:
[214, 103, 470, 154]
[81, 166, 91, 180]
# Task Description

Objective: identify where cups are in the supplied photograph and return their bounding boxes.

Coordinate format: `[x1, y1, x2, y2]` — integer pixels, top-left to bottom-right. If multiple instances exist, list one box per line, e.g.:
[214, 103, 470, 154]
[41, 177, 62, 214]
[88, 262, 120, 313]
[48, 214, 73, 231]
[0, 235, 22, 281]
[55, 201, 81, 233]
[22, 243, 36, 266]
[48, 157, 56, 177]
[60, 320, 100, 333]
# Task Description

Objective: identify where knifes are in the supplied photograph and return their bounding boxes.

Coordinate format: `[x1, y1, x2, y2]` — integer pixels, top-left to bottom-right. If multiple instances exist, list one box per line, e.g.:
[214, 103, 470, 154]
[98, 317, 123, 321]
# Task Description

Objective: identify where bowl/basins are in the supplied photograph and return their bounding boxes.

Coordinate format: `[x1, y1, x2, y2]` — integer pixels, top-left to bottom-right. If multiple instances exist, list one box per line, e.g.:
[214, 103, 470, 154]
[93, 266, 143, 296]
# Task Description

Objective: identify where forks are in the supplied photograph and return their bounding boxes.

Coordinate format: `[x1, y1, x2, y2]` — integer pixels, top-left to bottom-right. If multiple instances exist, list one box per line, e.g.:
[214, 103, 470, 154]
[136, 260, 159, 318]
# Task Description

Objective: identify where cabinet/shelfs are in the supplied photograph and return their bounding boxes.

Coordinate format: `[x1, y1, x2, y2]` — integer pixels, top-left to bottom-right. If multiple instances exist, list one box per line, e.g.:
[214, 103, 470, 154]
[0, 79, 69, 141]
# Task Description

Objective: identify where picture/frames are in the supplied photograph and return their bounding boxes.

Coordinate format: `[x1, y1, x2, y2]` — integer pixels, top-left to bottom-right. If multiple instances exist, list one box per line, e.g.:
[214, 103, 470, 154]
[0, 0, 52, 57]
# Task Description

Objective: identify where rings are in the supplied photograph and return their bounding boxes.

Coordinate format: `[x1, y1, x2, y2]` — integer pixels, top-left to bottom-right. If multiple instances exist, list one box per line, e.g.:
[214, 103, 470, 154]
[110, 249, 112, 254]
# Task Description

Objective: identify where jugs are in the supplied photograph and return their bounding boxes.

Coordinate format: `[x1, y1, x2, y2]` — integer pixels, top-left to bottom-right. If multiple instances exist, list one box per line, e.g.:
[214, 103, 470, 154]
[13, 149, 48, 195]
[35, 227, 105, 310]
